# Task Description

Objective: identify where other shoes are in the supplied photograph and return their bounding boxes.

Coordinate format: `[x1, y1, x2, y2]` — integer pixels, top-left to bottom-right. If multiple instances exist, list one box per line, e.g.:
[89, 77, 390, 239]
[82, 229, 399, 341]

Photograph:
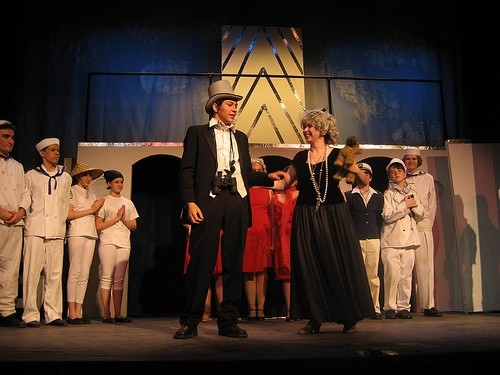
[102, 318, 113, 323]
[114, 316, 131, 323]
[66, 314, 91, 325]
[285, 316, 293, 322]
[202, 311, 211, 322]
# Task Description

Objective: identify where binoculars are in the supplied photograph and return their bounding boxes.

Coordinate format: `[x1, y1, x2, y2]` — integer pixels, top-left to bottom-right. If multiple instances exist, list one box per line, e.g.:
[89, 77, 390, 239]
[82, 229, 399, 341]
[212, 171, 237, 194]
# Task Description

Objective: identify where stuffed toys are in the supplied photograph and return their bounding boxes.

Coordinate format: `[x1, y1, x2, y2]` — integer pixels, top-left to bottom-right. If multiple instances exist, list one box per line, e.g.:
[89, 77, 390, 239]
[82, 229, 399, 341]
[333, 136, 362, 184]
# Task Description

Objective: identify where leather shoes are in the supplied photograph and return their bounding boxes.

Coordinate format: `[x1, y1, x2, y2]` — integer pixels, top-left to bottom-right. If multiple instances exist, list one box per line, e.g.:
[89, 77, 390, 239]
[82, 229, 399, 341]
[25, 320, 41, 328]
[218, 323, 248, 338]
[385, 310, 397, 319]
[0, 312, 28, 328]
[372, 312, 382, 319]
[47, 319, 66, 327]
[174, 324, 198, 339]
[423, 307, 442, 317]
[399, 309, 411, 319]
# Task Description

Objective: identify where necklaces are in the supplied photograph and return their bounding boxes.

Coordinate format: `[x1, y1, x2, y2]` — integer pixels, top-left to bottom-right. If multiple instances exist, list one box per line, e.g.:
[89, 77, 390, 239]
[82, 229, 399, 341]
[308, 144, 328, 210]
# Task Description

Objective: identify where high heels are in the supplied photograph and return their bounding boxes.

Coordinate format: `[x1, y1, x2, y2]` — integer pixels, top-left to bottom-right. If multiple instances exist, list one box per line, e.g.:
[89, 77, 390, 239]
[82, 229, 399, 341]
[297, 320, 321, 334]
[248, 314, 257, 322]
[257, 315, 265, 322]
[343, 321, 357, 333]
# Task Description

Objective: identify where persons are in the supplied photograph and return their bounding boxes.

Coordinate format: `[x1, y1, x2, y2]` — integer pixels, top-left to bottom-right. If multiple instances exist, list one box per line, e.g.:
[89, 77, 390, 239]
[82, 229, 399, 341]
[381, 158, 425, 320]
[260, 109, 377, 335]
[66, 162, 106, 325]
[180, 158, 300, 321]
[21, 138, 72, 329]
[174, 80, 289, 339]
[403, 149, 442, 317]
[344, 162, 384, 320]
[0, 120, 32, 328]
[95, 170, 139, 323]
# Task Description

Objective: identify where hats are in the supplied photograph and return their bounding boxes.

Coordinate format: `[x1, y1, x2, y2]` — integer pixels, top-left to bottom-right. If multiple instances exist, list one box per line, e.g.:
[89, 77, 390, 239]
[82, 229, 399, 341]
[386, 158, 407, 173]
[403, 147, 421, 157]
[356, 162, 372, 175]
[205, 80, 243, 114]
[70, 162, 104, 181]
[36, 137, 60, 152]
